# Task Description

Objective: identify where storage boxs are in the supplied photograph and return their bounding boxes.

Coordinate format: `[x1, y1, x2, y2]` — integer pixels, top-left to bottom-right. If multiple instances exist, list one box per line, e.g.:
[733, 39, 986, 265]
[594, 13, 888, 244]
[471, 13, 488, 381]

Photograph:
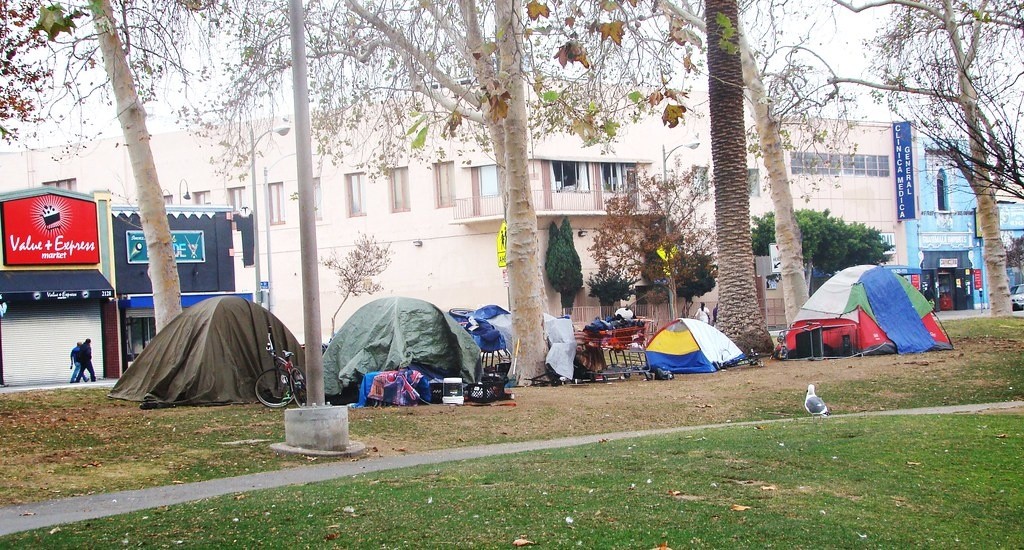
[428, 372, 509, 403]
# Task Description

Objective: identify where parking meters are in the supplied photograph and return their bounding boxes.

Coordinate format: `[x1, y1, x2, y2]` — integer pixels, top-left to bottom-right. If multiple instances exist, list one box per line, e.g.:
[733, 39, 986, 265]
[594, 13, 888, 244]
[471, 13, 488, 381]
[979, 288, 983, 313]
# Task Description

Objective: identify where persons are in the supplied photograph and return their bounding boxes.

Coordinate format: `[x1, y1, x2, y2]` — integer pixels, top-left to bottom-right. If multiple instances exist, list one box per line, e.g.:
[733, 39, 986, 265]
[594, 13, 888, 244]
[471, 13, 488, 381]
[76, 338, 96, 383]
[693, 302, 710, 324]
[713, 303, 718, 328]
[924, 286, 935, 312]
[70, 342, 89, 383]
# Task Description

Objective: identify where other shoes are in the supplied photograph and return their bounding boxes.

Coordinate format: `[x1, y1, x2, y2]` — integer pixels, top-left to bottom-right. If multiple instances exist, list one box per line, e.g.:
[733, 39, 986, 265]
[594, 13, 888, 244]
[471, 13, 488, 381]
[91, 380, 96, 382]
[85, 378, 89, 382]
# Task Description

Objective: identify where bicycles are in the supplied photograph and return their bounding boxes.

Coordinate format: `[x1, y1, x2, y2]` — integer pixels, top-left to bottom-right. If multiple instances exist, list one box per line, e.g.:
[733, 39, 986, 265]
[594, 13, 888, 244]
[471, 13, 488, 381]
[254, 325, 307, 409]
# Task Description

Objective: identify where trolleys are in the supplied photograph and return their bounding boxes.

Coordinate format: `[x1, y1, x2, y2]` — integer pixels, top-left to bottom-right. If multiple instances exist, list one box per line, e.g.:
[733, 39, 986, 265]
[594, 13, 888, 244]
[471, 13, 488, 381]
[777, 321, 863, 362]
[583, 317, 654, 382]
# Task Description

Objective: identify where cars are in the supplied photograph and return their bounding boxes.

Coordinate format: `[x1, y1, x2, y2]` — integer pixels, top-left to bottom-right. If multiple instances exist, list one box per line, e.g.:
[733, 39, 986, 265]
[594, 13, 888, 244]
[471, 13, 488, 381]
[1010, 284, 1024, 311]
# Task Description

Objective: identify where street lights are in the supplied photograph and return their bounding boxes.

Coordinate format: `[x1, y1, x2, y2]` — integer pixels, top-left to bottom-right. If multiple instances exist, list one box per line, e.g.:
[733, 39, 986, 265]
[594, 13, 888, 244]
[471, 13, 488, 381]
[662, 140, 701, 320]
[179, 179, 191, 205]
[249, 125, 290, 306]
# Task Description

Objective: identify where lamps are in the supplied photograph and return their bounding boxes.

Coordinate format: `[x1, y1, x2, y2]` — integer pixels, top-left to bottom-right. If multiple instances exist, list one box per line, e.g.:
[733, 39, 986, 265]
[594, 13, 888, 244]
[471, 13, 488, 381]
[413, 240, 423, 247]
[578, 229, 589, 236]
[178, 176, 192, 206]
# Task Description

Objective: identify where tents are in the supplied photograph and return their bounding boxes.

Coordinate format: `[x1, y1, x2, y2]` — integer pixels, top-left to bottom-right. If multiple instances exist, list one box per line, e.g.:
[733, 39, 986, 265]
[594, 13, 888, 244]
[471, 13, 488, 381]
[630, 318, 746, 373]
[323, 296, 482, 406]
[771, 265, 954, 360]
[107, 296, 307, 405]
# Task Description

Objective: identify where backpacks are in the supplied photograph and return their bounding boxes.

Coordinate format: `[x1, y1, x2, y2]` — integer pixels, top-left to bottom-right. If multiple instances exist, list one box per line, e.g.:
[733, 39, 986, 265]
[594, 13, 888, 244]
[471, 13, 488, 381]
[925, 290, 930, 301]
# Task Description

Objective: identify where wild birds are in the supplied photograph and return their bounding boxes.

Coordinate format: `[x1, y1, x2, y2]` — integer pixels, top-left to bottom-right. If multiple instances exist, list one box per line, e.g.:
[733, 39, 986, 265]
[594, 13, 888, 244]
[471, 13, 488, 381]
[804, 384, 832, 420]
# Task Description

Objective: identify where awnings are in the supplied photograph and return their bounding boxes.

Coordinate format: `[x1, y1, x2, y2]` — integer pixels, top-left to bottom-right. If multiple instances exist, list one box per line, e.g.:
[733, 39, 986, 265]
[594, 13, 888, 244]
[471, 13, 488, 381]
[0, 269, 115, 303]
